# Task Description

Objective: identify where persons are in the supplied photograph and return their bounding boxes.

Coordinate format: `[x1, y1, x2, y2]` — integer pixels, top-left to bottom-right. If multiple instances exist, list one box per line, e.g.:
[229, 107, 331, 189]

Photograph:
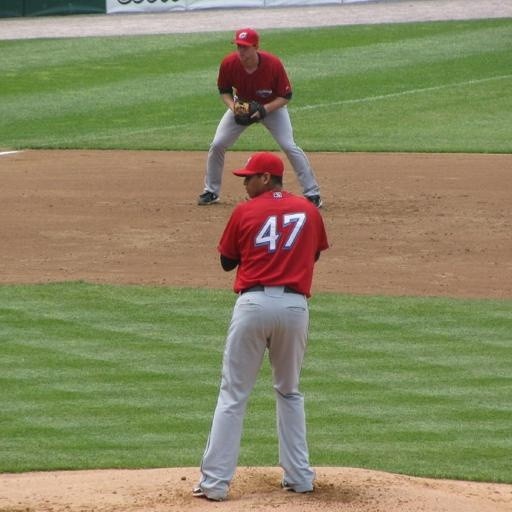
[190, 151, 329, 500]
[196, 27, 324, 210]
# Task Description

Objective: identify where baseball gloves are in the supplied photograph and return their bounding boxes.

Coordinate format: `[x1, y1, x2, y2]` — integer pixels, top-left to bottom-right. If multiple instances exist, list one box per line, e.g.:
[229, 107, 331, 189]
[234, 100, 266, 125]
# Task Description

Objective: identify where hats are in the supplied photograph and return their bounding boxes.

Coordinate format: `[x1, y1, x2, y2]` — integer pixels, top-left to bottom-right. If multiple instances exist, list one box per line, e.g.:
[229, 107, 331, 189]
[230, 28, 259, 47]
[232, 152, 284, 177]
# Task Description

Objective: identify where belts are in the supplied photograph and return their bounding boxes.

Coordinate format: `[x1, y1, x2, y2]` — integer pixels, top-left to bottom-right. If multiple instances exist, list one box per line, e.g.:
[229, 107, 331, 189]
[242, 284, 298, 293]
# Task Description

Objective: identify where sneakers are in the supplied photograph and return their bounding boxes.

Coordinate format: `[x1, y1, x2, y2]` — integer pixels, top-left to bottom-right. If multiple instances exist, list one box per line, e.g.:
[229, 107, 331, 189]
[307, 195, 324, 208]
[192, 485, 204, 496]
[280, 477, 294, 490]
[198, 190, 221, 205]
